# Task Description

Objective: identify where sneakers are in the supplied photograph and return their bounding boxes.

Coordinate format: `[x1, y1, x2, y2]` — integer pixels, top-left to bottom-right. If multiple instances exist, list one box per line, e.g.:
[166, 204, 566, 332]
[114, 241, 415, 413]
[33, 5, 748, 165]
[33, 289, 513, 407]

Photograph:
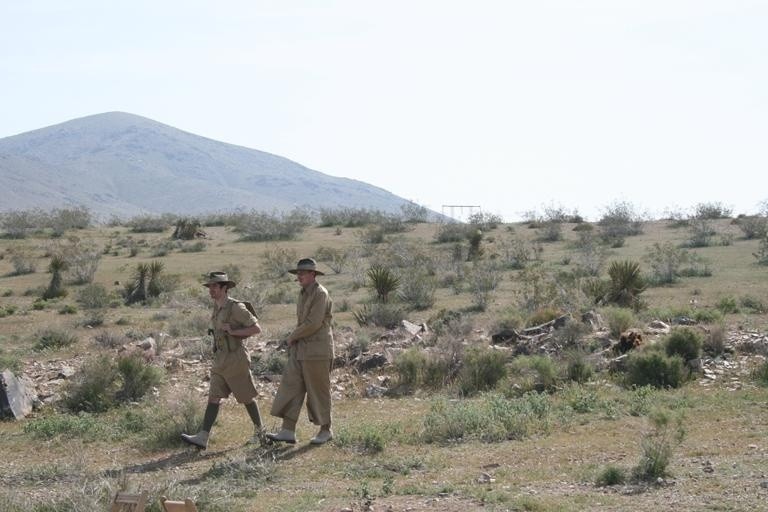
[310, 429, 333, 445]
[246, 424, 265, 444]
[181, 429, 210, 450]
[266, 428, 296, 444]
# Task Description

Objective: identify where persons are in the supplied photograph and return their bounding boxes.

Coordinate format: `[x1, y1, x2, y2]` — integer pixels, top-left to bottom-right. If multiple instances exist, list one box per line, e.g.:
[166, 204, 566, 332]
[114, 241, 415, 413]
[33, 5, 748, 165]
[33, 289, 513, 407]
[264, 258, 334, 446]
[181, 270, 267, 450]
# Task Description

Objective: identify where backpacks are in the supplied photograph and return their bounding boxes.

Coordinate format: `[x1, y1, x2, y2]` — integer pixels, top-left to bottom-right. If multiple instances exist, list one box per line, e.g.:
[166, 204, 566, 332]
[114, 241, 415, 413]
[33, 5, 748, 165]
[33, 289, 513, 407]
[211, 299, 259, 340]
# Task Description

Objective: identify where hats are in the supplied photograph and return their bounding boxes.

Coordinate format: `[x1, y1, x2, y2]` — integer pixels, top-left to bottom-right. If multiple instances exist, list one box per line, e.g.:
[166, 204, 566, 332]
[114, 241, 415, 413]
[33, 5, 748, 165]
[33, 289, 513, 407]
[288, 258, 325, 276]
[201, 271, 235, 289]
